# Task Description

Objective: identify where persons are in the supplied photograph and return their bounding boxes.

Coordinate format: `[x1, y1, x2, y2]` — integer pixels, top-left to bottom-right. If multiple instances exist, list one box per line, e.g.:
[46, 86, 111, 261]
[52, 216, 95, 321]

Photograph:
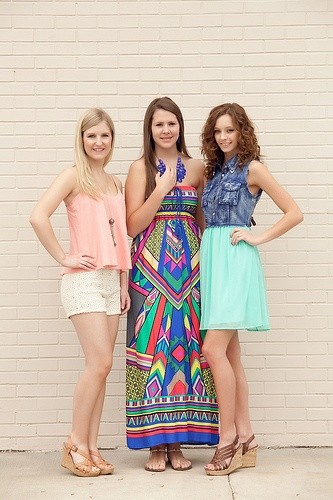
[192, 102, 304, 476]
[28, 106, 131, 479]
[124, 96, 210, 472]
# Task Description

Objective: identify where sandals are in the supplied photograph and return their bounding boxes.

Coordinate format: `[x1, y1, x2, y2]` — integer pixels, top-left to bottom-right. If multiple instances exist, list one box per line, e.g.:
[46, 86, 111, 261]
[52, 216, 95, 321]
[144, 446, 192, 472]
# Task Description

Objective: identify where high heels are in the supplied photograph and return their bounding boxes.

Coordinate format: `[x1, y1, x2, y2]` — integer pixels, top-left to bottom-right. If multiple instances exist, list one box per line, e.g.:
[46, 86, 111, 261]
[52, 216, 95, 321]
[61, 436, 114, 477]
[205, 433, 260, 476]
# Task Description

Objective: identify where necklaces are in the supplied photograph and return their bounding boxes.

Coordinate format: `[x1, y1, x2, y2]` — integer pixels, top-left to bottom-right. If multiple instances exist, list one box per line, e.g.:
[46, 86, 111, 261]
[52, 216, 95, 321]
[156, 153, 181, 177]
